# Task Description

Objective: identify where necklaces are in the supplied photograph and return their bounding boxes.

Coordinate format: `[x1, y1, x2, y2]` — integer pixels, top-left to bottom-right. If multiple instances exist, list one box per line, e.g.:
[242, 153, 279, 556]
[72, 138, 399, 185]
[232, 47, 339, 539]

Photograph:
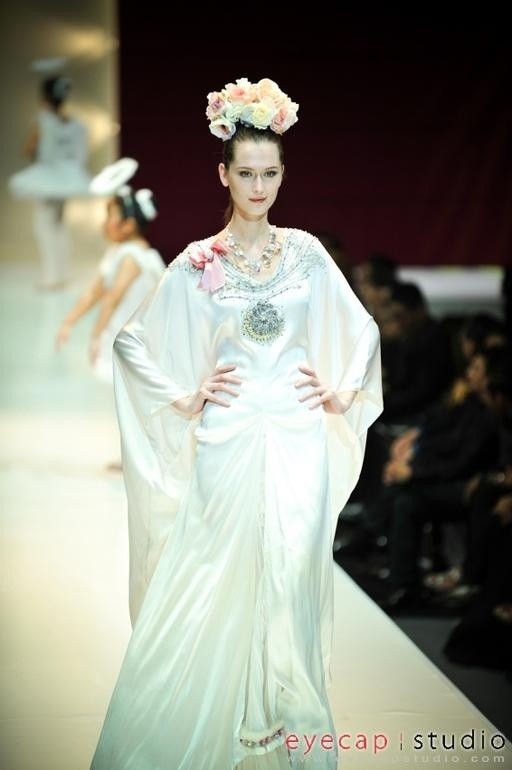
[224, 220, 285, 275]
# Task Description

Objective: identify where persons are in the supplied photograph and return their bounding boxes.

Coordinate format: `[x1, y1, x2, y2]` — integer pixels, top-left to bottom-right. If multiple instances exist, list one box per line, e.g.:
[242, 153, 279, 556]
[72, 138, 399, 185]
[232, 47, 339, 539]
[12, 71, 97, 279]
[322, 223, 510, 682]
[85, 76, 389, 770]
[53, 183, 168, 470]
